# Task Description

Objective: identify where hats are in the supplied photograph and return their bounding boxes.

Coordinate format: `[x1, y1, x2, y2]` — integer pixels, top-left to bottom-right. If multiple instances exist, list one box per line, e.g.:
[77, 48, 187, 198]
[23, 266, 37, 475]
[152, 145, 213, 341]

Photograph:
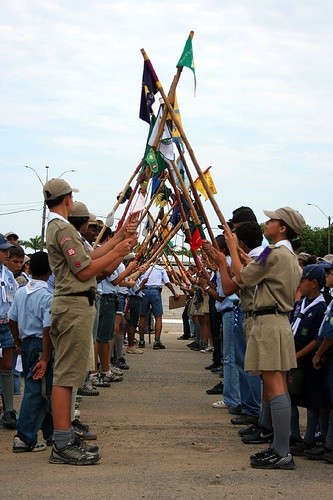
[217, 219, 234, 230]
[0, 233, 17, 249]
[4, 231, 19, 239]
[317, 261, 333, 268]
[262, 206, 307, 235]
[302, 263, 326, 282]
[88, 220, 100, 228]
[318, 254, 333, 263]
[68, 200, 96, 222]
[43, 178, 80, 199]
[124, 252, 136, 260]
[297, 252, 310, 261]
[21, 254, 30, 265]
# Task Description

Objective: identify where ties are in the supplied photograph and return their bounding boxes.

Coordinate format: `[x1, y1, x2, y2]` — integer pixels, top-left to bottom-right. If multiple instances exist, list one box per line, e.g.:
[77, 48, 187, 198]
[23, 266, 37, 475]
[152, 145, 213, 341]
[318, 300, 333, 336]
[292, 292, 325, 335]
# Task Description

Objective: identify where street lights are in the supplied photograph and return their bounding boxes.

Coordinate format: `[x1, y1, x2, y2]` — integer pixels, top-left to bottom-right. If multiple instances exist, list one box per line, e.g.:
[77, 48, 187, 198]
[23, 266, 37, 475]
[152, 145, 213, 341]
[307, 202, 332, 253]
[25, 163, 80, 254]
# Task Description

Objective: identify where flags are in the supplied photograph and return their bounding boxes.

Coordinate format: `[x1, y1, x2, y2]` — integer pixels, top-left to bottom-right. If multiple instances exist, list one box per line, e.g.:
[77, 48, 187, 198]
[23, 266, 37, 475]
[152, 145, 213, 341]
[136, 89, 205, 207]
[176, 35, 197, 97]
[139, 54, 162, 124]
[193, 170, 218, 201]
[142, 216, 154, 238]
[189, 228, 203, 250]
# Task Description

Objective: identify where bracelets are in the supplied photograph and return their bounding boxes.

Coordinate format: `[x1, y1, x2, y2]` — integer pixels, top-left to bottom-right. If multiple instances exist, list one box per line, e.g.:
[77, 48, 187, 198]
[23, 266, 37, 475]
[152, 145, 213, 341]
[203, 285, 210, 292]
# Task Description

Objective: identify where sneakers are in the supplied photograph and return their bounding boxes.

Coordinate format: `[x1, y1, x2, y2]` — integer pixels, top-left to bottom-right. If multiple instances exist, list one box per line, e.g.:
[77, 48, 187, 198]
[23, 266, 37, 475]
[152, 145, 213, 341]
[176, 333, 333, 469]
[2, 329, 166, 466]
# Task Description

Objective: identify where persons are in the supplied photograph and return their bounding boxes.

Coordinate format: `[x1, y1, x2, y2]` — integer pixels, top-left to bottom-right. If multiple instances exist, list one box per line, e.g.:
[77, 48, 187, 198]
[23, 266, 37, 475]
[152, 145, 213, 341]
[0, 201, 149, 460]
[199, 234, 246, 409]
[43, 178, 138, 466]
[181, 207, 333, 464]
[221, 207, 307, 470]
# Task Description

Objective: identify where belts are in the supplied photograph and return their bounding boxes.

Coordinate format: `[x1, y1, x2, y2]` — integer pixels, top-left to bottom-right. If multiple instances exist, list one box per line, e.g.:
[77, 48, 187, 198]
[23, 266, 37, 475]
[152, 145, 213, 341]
[254, 305, 283, 317]
[222, 306, 234, 314]
[144, 286, 158, 290]
[243, 310, 253, 318]
[101, 293, 115, 298]
[64, 291, 89, 296]
